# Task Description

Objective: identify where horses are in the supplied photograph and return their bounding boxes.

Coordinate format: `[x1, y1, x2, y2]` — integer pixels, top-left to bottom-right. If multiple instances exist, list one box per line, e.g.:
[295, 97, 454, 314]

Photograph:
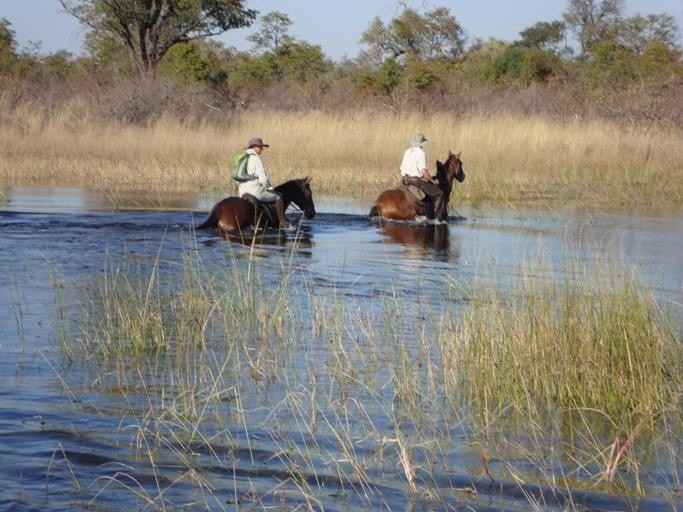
[370, 149, 465, 220]
[198, 176, 316, 232]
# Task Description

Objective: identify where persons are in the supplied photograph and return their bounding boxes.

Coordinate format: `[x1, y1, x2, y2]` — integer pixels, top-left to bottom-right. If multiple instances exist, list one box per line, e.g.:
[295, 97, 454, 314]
[398, 132, 449, 226]
[229, 137, 297, 232]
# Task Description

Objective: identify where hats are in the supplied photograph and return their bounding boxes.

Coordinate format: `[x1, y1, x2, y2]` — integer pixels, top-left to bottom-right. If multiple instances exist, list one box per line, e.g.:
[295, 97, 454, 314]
[248, 138, 270, 148]
[410, 133, 429, 147]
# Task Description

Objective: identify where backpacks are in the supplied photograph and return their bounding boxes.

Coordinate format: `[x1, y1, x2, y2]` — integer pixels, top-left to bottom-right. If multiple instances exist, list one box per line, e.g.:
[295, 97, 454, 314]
[231, 151, 258, 182]
